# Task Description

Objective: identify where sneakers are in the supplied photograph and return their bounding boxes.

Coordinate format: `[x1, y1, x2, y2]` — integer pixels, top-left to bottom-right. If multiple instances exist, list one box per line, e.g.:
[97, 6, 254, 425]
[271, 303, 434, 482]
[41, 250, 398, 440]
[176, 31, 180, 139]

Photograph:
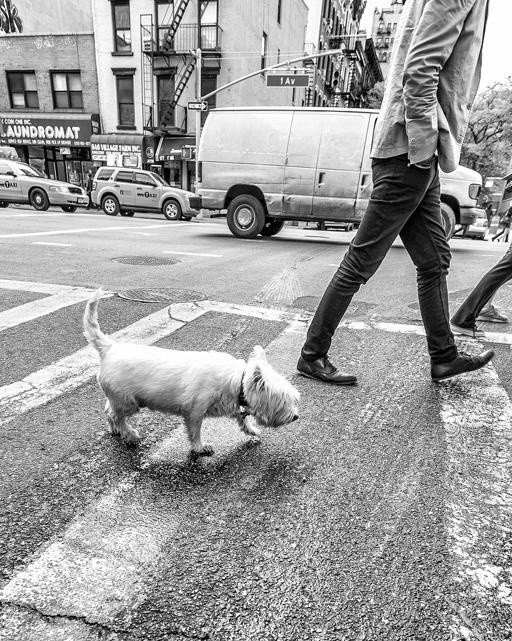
[475, 305, 508, 323]
[451, 323, 486, 337]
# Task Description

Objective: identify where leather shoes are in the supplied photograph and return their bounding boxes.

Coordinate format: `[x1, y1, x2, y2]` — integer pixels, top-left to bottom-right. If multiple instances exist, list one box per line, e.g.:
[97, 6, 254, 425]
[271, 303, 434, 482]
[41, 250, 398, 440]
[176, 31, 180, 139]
[297, 356, 358, 385]
[430, 350, 495, 381]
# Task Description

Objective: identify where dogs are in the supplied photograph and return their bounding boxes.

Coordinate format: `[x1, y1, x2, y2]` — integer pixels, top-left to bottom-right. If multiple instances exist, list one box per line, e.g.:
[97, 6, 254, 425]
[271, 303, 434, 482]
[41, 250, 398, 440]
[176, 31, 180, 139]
[81, 286, 301, 457]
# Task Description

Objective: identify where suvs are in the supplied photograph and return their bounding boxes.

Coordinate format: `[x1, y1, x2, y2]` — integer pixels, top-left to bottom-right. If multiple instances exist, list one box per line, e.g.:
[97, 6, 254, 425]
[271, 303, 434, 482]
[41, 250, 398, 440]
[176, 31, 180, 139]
[90, 167, 201, 221]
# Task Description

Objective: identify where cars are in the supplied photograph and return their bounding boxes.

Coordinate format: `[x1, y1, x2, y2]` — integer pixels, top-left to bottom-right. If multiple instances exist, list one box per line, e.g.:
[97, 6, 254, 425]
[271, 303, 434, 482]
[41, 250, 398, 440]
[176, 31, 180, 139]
[0, 159, 90, 213]
[454, 209, 488, 239]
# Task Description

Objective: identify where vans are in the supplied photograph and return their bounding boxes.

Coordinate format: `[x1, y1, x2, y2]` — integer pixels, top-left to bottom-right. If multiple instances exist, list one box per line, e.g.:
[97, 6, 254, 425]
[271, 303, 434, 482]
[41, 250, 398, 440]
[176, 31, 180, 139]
[198, 106, 482, 242]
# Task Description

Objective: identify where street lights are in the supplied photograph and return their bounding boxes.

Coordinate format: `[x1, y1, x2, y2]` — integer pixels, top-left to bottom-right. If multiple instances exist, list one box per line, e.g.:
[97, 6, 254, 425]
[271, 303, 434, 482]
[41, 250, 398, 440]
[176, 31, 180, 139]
[188, 48, 362, 183]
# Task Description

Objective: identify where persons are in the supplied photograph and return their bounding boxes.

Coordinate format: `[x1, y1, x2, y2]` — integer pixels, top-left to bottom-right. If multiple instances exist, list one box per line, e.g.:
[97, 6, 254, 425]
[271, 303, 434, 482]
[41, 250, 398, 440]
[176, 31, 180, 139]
[484, 203, 499, 228]
[492, 207, 511, 243]
[449, 240, 510, 337]
[86, 174, 100, 211]
[475, 289, 508, 321]
[297, 0, 495, 387]
